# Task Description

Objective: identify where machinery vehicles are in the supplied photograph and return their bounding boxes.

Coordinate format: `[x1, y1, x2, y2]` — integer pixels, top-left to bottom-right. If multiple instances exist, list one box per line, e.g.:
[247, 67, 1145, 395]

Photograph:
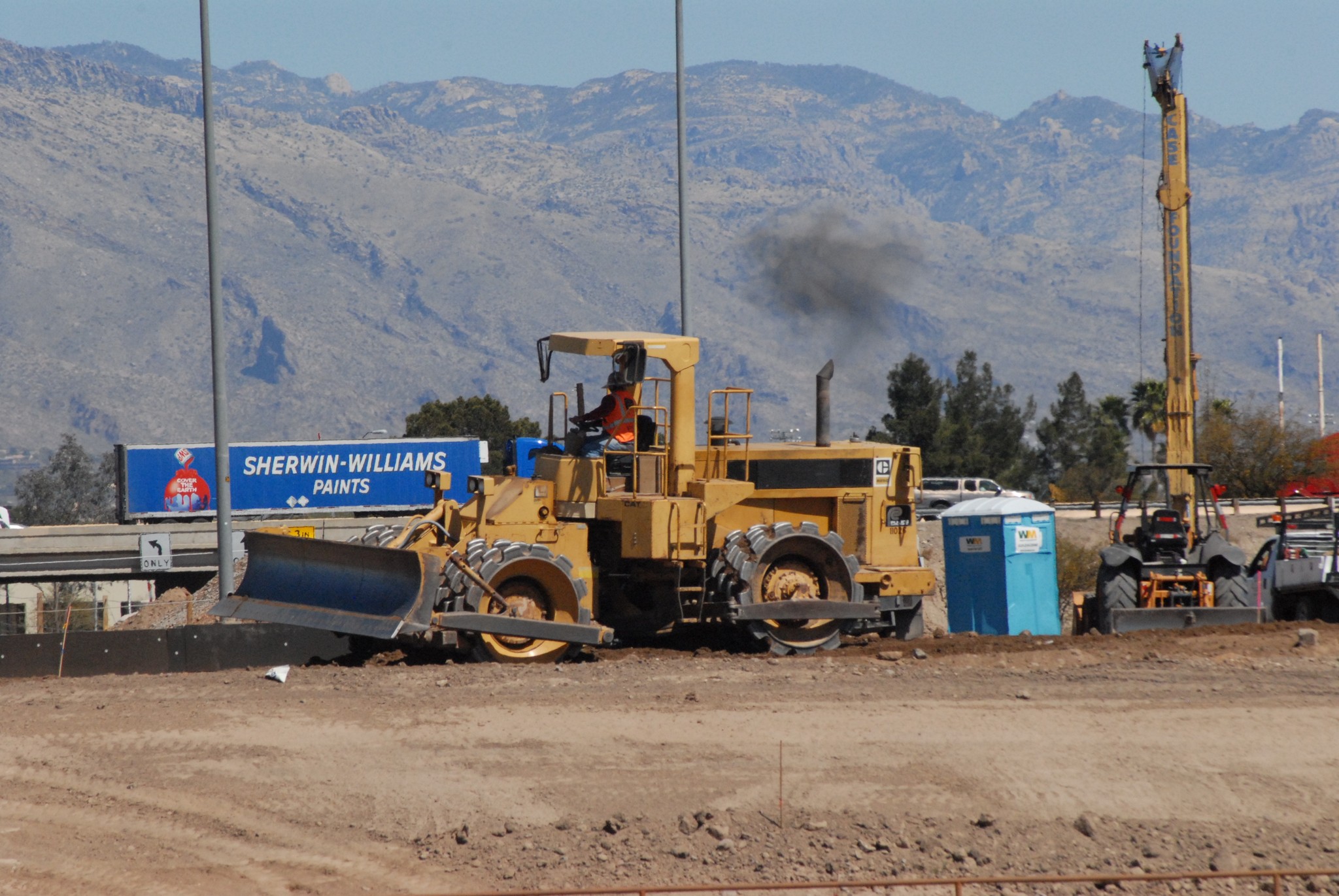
[1247, 432, 1338, 623]
[1071, 32, 1267, 639]
[204, 328, 935, 668]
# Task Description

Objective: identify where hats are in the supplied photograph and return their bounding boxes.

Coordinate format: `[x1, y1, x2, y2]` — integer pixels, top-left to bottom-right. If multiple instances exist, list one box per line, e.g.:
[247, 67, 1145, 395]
[601, 370, 632, 389]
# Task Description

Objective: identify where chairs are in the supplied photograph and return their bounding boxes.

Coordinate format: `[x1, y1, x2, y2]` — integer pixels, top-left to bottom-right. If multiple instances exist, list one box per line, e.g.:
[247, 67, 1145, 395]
[1143, 510, 1189, 549]
[606, 415, 654, 475]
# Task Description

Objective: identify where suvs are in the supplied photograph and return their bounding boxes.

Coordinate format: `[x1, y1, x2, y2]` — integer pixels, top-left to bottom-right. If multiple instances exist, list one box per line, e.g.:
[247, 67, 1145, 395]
[914, 476, 1036, 521]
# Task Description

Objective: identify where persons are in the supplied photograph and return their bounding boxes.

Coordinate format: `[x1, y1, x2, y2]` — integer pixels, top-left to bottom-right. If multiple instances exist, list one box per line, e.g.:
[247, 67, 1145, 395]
[570, 371, 639, 495]
